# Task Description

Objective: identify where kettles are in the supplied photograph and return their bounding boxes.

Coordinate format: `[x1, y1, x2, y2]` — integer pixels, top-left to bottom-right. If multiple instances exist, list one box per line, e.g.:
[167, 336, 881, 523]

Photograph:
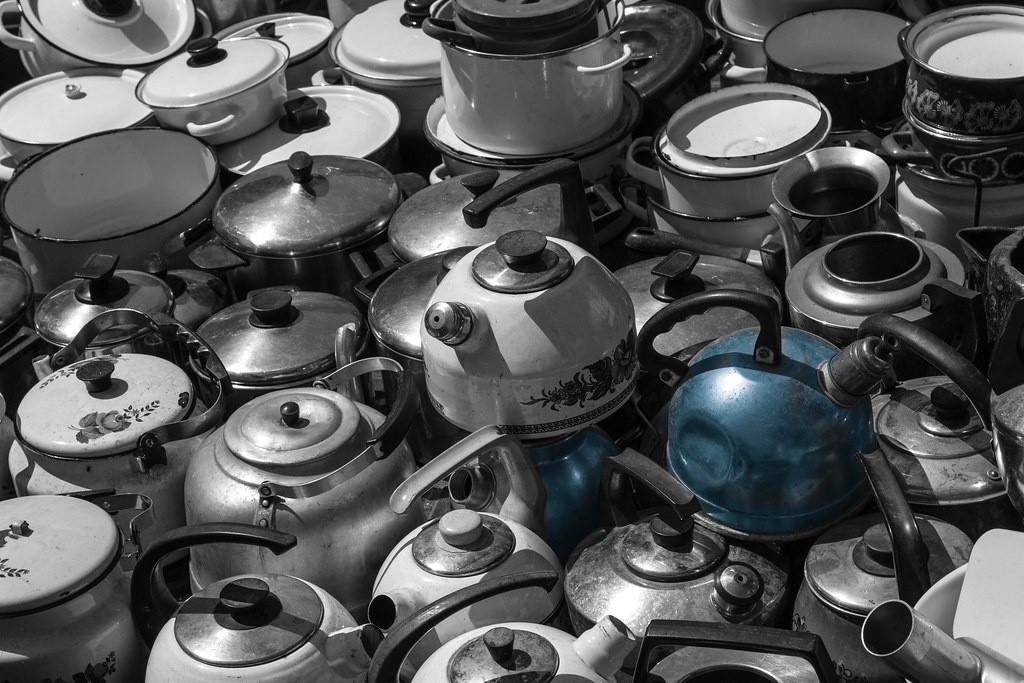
[0, 0, 1024, 683]
